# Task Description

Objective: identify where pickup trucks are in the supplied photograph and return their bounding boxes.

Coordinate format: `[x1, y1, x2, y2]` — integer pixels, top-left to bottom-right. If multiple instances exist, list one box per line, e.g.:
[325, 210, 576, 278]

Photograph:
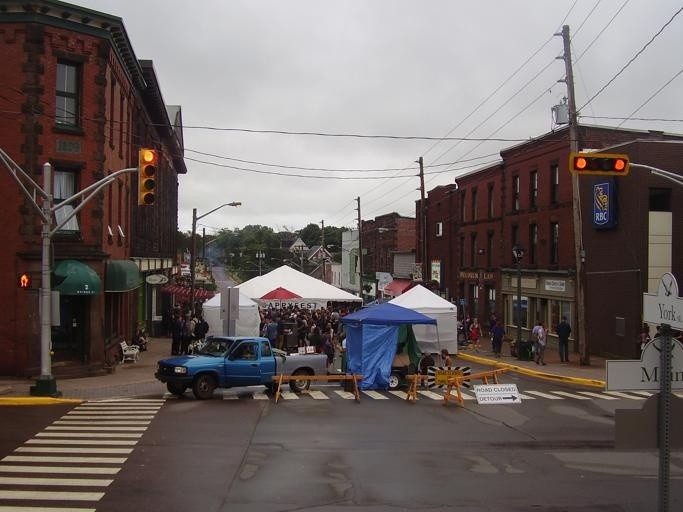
[154, 335, 330, 401]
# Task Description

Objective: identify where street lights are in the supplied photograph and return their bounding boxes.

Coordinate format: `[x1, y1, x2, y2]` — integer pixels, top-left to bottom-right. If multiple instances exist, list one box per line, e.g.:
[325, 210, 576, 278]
[190, 200, 243, 317]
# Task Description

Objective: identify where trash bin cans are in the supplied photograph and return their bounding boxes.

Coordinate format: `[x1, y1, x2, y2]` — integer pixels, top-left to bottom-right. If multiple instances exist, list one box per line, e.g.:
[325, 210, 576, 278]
[510, 338, 535, 361]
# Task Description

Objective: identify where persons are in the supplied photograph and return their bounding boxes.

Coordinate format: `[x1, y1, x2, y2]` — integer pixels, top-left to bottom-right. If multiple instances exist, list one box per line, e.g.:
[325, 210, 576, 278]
[130, 301, 209, 357]
[416, 350, 434, 387]
[257, 302, 359, 354]
[488, 313, 496, 353]
[490, 320, 505, 356]
[461, 316, 471, 342]
[456, 318, 463, 336]
[531, 320, 548, 366]
[438, 347, 452, 368]
[469, 317, 483, 352]
[637, 327, 650, 352]
[555, 315, 571, 362]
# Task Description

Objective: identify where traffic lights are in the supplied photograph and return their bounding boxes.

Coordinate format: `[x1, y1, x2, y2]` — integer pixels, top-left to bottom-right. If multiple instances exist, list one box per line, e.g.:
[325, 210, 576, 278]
[16, 271, 32, 289]
[137, 147, 159, 208]
[568, 151, 630, 176]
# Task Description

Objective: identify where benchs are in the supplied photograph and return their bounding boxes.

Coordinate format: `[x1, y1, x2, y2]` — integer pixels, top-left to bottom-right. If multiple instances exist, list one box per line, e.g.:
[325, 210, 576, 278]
[119, 340, 140, 364]
